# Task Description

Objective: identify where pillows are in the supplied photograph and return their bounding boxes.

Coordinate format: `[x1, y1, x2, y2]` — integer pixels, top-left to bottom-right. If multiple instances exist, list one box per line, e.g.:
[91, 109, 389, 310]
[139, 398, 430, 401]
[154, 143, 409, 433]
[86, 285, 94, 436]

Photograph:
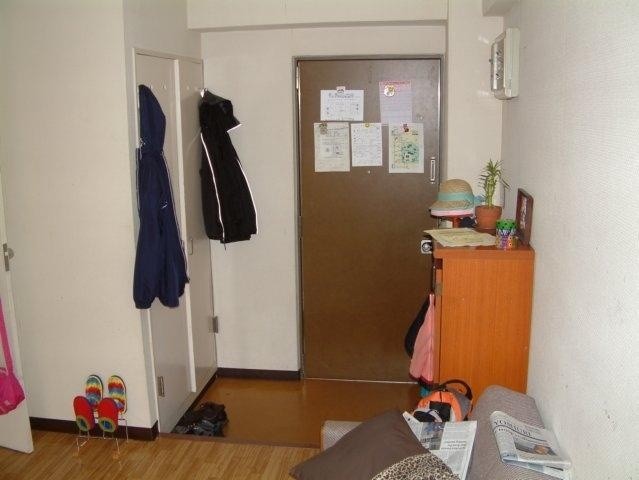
[288, 404, 460, 479]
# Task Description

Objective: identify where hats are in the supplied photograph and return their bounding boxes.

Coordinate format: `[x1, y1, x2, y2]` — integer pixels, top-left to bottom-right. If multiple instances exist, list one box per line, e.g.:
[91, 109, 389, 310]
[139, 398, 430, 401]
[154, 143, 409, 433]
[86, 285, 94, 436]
[428, 179, 485, 211]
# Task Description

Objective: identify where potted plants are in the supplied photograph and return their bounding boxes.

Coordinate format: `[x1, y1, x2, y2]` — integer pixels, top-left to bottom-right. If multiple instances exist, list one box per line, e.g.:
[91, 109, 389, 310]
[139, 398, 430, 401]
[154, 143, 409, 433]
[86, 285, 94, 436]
[475, 157, 510, 229]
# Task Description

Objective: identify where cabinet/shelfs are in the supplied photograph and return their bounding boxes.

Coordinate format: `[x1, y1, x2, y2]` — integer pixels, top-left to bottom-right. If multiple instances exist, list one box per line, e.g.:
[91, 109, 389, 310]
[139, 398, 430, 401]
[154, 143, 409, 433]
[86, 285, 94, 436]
[431, 227, 535, 421]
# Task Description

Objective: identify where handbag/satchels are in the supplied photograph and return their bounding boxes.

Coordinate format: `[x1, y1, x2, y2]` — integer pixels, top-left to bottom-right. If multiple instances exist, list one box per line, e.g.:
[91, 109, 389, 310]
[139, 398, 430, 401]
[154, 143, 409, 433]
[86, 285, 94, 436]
[0, 367, 25, 415]
[411, 379, 473, 422]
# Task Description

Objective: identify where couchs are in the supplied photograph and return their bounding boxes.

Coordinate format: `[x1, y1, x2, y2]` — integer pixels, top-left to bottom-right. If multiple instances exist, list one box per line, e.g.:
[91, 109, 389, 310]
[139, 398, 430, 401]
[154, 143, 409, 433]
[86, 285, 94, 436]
[318, 385, 564, 480]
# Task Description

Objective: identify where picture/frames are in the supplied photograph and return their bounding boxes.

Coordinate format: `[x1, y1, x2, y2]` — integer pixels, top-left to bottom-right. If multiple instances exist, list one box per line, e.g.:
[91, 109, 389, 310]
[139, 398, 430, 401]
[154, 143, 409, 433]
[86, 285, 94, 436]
[514, 188, 533, 246]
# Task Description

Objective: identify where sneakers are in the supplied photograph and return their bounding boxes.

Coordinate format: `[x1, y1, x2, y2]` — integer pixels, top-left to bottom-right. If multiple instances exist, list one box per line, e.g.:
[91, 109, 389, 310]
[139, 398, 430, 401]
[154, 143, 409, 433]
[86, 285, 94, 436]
[171, 401, 229, 437]
[108, 376, 127, 415]
[85, 375, 102, 411]
[97, 398, 118, 433]
[73, 396, 95, 432]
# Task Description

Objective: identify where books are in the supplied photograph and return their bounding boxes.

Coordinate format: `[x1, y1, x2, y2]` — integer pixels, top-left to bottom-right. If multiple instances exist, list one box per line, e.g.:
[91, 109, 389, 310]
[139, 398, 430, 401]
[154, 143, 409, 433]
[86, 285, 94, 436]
[423, 224, 499, 248]
